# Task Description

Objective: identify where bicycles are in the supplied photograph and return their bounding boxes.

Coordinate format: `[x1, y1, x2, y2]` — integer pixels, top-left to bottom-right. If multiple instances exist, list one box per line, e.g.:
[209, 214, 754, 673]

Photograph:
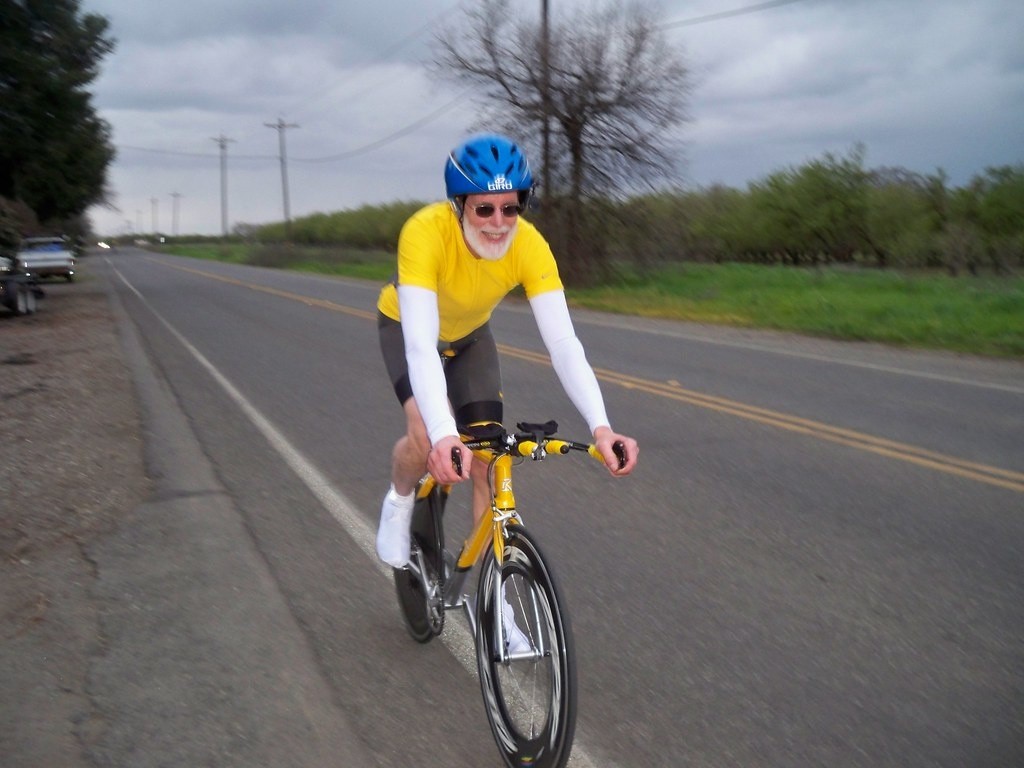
[393, 350, 627, 768]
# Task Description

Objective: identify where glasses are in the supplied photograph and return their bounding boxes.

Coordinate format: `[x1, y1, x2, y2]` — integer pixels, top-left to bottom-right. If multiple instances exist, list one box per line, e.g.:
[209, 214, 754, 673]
[462, 198, 522, 217]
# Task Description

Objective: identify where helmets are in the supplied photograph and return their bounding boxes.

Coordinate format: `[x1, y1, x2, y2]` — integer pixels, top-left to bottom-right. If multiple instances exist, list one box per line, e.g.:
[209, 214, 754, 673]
[444, 133, 532, 199]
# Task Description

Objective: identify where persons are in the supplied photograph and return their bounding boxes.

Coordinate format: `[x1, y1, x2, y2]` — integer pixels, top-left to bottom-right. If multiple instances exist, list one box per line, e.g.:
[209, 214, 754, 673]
[378, 139, 640, 659]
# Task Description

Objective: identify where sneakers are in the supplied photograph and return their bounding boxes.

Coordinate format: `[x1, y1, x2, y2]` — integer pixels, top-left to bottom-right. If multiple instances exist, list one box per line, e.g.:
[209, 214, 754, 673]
[474, 595, 531, 655]
[375, 491, 412, 568]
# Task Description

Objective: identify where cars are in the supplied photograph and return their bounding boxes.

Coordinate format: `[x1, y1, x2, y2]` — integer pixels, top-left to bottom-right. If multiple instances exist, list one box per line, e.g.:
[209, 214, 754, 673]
[0, 226, 76, 315]
